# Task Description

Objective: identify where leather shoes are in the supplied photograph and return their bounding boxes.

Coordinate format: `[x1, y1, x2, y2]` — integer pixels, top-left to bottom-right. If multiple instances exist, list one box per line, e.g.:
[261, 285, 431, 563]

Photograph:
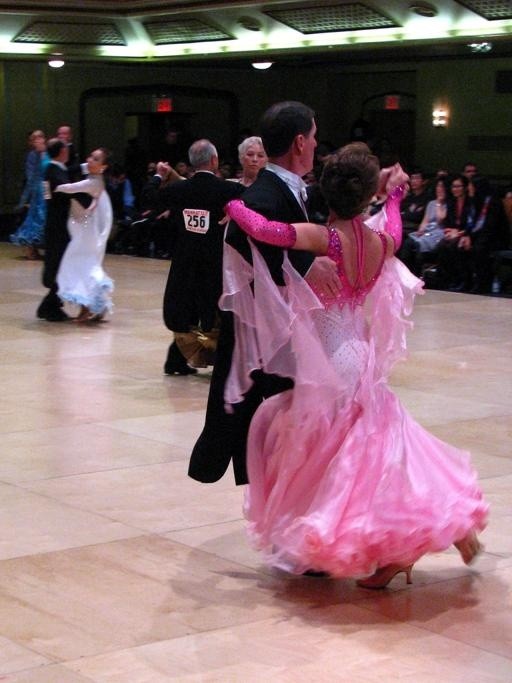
[164, 362, 197, 375]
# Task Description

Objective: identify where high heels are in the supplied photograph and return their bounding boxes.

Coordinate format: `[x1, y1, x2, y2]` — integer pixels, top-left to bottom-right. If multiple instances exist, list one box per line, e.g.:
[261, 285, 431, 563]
[356, 559, 414, 590]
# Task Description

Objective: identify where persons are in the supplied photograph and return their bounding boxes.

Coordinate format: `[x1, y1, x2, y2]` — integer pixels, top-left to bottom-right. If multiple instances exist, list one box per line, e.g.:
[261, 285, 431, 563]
[143, 135, 268, 374]
[218, 142, 491, 590]
[104, 128, 511, 298]
[187, 100, 410, 577]
[36, 140, 115, 323]
[9, 126, 78, 260]
